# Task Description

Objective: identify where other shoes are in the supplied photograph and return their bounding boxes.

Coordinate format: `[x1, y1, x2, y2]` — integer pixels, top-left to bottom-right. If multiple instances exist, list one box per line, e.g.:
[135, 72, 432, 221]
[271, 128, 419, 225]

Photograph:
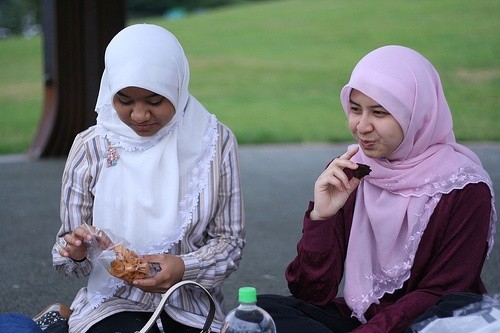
[32, 303, 72, 330]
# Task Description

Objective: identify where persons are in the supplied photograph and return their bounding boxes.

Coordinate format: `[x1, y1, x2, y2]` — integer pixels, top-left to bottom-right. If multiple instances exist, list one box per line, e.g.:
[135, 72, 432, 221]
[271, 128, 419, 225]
[256, 46, 500, 333]
[33, 24, 246, 333]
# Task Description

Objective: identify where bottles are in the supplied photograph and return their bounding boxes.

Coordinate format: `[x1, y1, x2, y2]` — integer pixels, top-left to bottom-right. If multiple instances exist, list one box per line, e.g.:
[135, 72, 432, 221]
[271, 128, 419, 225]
[220, 287, 276, 333]
